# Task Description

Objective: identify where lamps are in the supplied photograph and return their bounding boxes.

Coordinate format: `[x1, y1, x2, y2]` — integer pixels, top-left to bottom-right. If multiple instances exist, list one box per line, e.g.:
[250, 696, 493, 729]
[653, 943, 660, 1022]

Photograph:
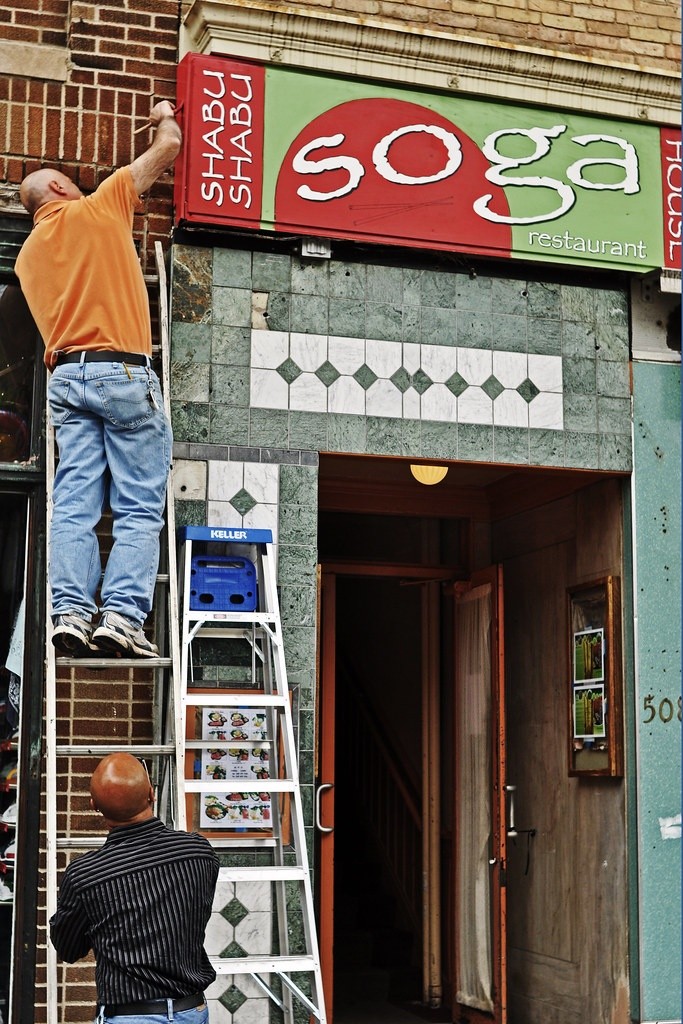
[410, 464, 449, 486]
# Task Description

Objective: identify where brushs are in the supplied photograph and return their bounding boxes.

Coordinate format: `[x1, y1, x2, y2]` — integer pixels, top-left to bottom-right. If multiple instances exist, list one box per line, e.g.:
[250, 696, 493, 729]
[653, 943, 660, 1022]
[132, 102, 185, 134]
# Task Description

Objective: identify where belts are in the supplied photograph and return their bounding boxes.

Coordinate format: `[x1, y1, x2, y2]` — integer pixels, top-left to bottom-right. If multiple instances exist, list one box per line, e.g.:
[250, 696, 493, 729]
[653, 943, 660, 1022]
[95, 992, 204, 1018]
[55, 351, 147, 366]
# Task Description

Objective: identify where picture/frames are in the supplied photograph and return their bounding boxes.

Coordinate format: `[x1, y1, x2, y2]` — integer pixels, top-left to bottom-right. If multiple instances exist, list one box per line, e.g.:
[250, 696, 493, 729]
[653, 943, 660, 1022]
[186, 688, 293, 846]
[573, 628, 604, 684]
[574, 684, 606, 738]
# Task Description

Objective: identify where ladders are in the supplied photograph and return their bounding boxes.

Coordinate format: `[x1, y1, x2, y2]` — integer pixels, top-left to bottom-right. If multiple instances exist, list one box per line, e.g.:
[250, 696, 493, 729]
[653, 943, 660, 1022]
[167, 525, 328, 1024]
[44, 240, 185, 1024]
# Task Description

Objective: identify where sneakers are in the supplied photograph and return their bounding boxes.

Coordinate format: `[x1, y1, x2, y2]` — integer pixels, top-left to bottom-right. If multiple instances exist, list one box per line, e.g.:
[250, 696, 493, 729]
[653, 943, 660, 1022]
[92, 611, 160, 657]
[51, 615, 107, 671]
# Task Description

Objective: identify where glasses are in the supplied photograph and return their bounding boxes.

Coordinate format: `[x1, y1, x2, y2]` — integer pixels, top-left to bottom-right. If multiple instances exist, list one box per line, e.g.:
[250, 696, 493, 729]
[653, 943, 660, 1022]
[135, 756, 152, 800]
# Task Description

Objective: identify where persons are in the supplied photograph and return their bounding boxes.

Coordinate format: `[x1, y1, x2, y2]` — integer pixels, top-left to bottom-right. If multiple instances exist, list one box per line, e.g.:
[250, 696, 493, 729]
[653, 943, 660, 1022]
[49, 752, 220, 1024]
[13, 99, 183, 659]
